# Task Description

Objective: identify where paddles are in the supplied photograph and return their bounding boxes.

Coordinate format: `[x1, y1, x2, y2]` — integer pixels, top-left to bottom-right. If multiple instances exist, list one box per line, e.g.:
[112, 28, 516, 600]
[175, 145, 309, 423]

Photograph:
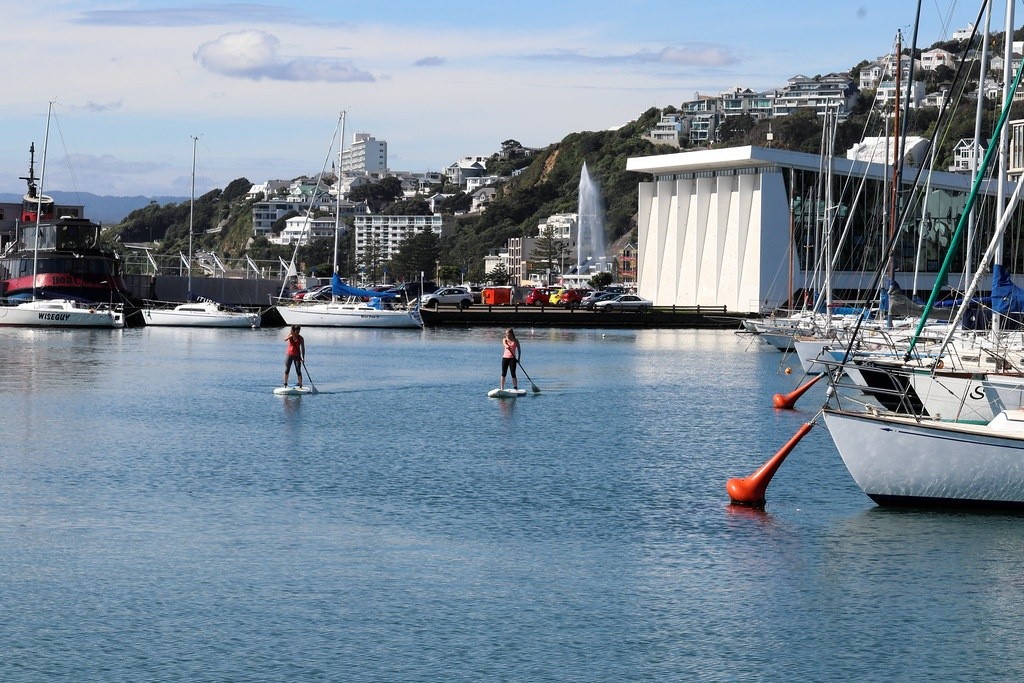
[289, 336, 320, 396]
[506, 346, 542, 395]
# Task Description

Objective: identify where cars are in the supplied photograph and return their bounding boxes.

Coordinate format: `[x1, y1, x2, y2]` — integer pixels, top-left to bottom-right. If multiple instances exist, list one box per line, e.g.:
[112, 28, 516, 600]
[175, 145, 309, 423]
[454, 283, 655, 313]
[357, 283, 395, 303]
[289, 284, 333, 304]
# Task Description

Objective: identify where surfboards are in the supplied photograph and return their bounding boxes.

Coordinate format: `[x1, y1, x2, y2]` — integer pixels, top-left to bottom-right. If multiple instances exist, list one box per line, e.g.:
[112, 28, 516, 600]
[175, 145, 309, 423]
[273, 385, 310, 395]
[487, 388, 527, 399]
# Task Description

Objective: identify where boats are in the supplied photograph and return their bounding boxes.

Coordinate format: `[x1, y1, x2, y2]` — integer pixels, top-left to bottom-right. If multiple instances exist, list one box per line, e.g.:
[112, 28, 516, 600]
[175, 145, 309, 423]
[0, 140, 126, 304]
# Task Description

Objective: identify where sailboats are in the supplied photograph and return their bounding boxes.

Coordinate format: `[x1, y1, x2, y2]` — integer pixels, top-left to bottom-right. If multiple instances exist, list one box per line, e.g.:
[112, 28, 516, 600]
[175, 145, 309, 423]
[140, 133, 262, 328]
[267, 109, 425, 330]
[743, 0, 1024, 518]
[0, 97, 127, 328]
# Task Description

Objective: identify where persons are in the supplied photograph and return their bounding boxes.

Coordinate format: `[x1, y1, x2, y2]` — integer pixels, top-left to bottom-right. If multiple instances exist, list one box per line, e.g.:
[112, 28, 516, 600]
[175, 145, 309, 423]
[500, 328, 521, 389]
[283, 324, 305, 388]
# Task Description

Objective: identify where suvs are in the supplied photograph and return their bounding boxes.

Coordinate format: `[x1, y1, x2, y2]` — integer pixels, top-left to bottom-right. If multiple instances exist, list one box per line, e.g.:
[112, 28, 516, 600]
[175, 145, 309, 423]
[382, 280, 440, 303]
[420, 287, 475, 309]
